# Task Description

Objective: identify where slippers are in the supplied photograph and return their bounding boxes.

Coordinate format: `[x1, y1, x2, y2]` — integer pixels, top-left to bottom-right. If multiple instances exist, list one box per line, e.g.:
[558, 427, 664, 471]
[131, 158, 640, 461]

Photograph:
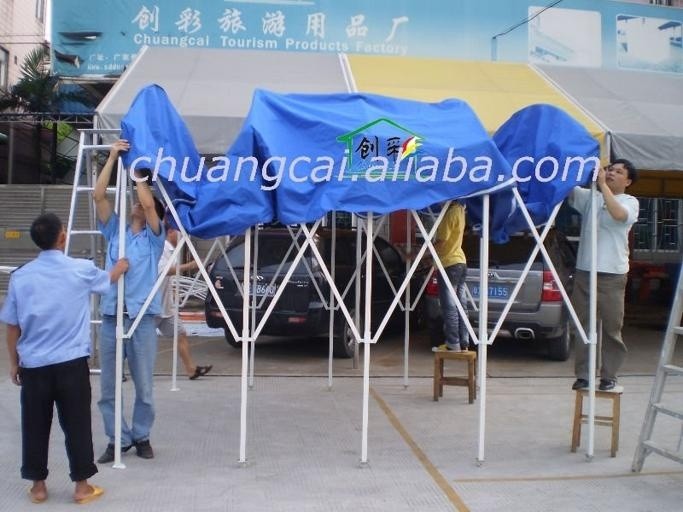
[72, 483, 105, 505]
[25, 486, 46, 504]
[188, 361, 213, 379]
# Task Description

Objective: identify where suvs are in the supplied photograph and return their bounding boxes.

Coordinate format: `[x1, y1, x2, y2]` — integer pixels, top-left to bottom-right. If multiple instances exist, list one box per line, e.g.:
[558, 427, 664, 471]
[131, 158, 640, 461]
[423, 228, 577, 361]
[203, 225, 422, 359]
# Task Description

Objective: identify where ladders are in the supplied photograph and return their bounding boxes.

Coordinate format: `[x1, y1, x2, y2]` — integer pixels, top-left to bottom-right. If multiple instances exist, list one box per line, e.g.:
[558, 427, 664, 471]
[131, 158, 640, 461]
[632, 261, 683, 474]
[48, 128, 128, 469]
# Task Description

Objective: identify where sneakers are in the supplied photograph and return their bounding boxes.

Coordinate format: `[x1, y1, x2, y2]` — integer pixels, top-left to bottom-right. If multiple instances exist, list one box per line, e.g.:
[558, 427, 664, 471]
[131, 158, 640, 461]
[430, 343, 473, 353]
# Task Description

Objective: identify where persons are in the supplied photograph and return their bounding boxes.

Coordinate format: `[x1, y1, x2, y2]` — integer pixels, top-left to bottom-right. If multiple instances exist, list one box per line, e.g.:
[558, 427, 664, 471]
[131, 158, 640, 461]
[92, 139, 166, 464]
[409, 199, 470, 353]
[568, 158, 640, 390]
[123, 224, 212, 382]
[0, 212, 128, 505]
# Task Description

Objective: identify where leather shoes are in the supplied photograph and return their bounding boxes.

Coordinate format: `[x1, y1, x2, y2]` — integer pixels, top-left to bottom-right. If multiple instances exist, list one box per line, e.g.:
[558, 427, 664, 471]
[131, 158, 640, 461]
[134, 438, 156, 459]
[95, 440, 133, 465]
[569, 377, 590, 390]
[596, 379, 616, 391]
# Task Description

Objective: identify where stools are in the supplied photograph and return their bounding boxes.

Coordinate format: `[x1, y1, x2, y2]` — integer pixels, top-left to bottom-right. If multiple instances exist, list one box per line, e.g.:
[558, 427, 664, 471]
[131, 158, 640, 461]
[433, 351, 477, 404]
[571, 385, 624, 457]
